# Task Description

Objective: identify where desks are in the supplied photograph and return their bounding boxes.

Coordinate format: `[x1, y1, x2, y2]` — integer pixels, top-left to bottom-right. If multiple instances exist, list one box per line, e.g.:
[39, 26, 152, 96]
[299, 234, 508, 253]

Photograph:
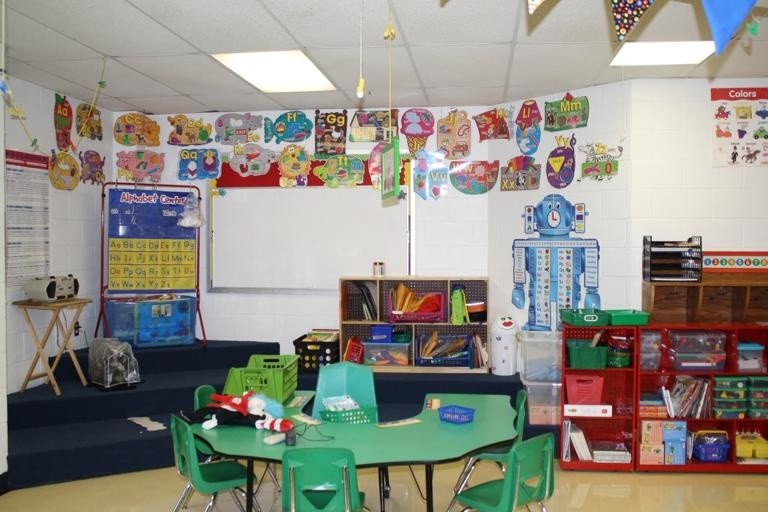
[11, 297, 92, 396]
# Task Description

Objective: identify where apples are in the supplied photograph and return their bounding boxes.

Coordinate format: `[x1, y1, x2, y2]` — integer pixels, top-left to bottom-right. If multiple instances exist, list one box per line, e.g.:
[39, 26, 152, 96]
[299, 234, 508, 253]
[715, 105, 731, 120]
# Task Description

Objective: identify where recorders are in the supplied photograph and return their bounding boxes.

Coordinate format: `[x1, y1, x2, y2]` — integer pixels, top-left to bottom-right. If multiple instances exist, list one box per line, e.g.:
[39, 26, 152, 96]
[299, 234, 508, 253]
[24, 273, 80, 302]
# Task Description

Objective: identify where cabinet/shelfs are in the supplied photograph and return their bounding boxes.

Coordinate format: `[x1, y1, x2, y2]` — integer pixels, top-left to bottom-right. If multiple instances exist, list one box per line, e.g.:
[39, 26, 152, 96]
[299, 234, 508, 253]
[337, 276, 491, 375]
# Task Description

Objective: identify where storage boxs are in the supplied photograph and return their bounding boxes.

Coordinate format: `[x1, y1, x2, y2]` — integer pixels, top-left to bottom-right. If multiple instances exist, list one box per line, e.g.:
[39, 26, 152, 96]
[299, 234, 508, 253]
[105, 296, 197, 349]
[517, 329, 562, 426]
[360, 337, 412, 366]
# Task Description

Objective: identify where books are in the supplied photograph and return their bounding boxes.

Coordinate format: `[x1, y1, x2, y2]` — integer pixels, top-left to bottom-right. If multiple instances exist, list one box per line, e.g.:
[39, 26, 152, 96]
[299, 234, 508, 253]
[569, 424, 631, 465]
[685, 431, 694, 461]
[376, 417, 422, 428]
[333, 401, 369, 424]
[418, 328, 488, 369]
[288, 412, 326, 426]
[286, 393, 306, 410]
[562, 419, 570, 462]
[320, 395, 370, 424]
[639, 375, 710, 419]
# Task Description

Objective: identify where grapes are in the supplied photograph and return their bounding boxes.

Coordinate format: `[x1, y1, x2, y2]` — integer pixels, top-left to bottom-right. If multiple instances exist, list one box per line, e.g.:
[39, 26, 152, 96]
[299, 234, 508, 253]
[737, 129, 747, 139]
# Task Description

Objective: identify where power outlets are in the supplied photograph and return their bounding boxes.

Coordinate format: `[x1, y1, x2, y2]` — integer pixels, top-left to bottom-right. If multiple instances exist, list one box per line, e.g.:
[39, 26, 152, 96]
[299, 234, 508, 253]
[73, 322, 82, 337]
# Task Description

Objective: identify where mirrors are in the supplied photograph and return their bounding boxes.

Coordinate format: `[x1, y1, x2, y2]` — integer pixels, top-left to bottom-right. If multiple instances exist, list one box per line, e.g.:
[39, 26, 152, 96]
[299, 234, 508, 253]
[380, 137, 401, 201]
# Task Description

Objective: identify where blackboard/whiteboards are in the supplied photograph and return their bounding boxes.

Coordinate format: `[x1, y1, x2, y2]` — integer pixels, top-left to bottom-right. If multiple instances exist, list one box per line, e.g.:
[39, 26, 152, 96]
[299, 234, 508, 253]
[207, 148, 411, 293]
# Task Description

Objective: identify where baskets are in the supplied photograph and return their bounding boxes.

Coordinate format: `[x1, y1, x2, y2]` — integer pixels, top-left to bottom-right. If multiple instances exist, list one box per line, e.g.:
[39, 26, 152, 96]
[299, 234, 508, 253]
[319, 404, 378, 424]
[693, 443, 731, 462]
[293, 334, 339, 372]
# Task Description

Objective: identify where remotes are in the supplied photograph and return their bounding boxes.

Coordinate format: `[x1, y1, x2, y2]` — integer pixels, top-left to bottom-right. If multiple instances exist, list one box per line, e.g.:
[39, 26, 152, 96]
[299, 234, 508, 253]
[286, 430, 296, 446]
[264, 433, 286, 444]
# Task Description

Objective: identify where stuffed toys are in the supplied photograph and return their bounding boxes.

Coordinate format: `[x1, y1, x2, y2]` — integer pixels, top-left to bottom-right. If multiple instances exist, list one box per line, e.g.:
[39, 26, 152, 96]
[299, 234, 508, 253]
[206, 391, 267, 418]
[177, 405, 294, 436]
